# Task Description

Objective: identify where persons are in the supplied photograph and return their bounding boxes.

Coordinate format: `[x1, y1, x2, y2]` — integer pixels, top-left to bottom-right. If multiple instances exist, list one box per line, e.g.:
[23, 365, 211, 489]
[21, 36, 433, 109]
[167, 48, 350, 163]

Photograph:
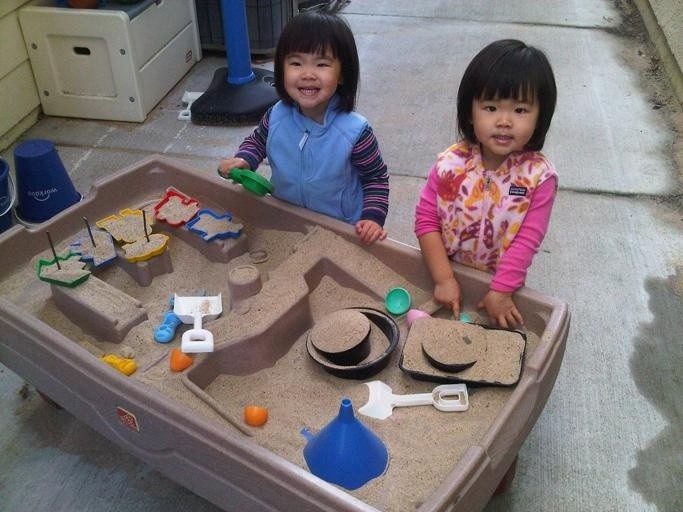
[414, 38, 559, 332]
[217, 10, 390, 248]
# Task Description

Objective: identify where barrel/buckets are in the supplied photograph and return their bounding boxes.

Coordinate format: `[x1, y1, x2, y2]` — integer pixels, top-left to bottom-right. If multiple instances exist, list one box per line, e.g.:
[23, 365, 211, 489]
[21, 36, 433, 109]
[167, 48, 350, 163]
[14, 139, 87, 230]
[0, 156, 17, 234]
